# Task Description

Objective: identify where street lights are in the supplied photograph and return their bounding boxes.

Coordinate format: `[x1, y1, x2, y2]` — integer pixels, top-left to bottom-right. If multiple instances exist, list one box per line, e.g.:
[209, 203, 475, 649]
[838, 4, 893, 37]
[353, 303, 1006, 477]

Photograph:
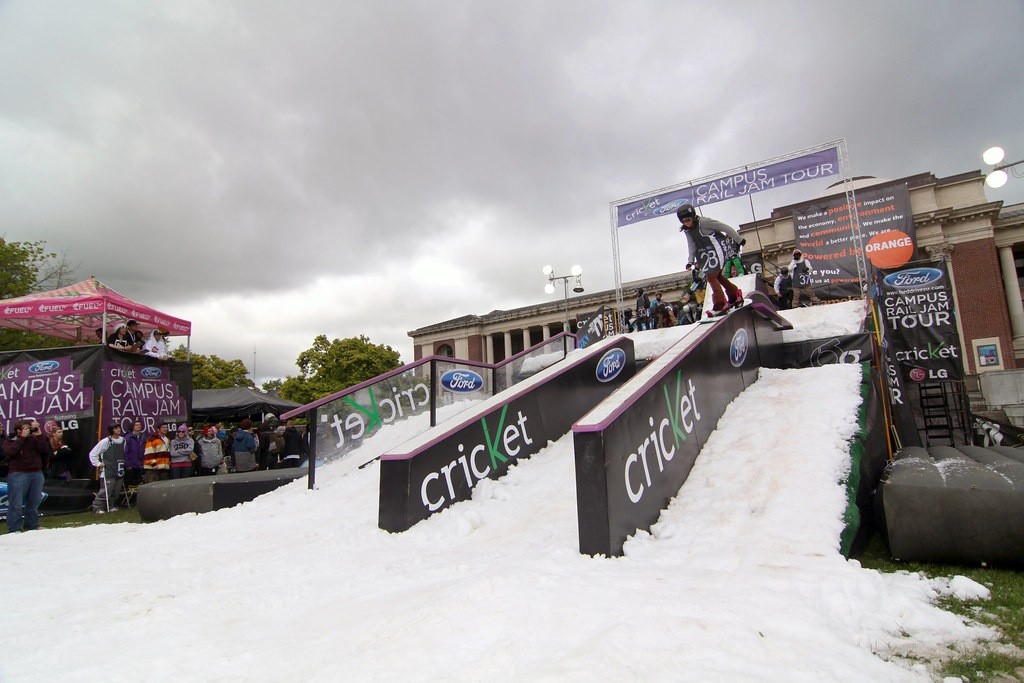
[550, 274, 584, 330]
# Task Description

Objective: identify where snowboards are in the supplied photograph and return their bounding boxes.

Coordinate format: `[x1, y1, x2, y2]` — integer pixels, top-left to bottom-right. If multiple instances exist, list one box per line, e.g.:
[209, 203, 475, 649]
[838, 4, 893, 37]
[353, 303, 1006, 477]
[697, 298, 753, 325]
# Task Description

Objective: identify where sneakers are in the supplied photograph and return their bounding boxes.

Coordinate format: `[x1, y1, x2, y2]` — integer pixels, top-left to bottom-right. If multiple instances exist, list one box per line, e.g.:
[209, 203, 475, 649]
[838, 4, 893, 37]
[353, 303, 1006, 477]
[725, 289, 742, 307]
[705, 302, 729, 317]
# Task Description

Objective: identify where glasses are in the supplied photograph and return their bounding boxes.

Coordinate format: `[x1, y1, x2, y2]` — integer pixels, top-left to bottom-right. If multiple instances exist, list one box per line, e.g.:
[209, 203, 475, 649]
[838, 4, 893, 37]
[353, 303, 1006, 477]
[188, 428, 194, 431]
[178, 431, 185, 433]
[682, 218, 691, 223]
[121, 326, 128, 329]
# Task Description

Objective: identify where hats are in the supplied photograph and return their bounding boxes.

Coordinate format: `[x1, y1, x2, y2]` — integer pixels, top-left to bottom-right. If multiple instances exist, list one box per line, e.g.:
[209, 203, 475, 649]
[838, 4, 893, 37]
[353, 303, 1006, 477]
[127, 320, 140, 325]
[655, 291, 663, 297]
[178, 423, 188, 435]
[203, 427, 214, 435]
[240, 419, 253, 430]
[113, 323, 127, 332]
[154, 330, 163, 335]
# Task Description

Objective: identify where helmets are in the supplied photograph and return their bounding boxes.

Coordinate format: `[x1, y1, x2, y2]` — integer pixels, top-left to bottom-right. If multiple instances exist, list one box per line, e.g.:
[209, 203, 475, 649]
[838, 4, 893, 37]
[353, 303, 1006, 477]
[781, 267, 788, 274]
[677, 204, 695, 222]
[107, 422, 123, 435]
[792, 249, 802, 256]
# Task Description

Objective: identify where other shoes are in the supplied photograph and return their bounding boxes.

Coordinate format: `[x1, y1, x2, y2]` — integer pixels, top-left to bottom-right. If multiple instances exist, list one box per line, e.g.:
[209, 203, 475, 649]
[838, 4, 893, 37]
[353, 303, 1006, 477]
[110, 507, 118, 512]
[96, 509, 105, 514]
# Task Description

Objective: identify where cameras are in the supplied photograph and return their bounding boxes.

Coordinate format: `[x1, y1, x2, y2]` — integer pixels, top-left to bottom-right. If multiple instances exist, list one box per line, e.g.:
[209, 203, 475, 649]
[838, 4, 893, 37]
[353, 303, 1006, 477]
[28, 427, 38, 434]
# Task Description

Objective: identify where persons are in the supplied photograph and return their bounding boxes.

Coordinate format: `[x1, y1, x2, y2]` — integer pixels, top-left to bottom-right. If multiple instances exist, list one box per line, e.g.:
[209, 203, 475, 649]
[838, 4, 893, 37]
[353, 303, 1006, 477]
[618, 249, 823, 334]
[0, 412, 311, 533]
[677, 204, 746, 318]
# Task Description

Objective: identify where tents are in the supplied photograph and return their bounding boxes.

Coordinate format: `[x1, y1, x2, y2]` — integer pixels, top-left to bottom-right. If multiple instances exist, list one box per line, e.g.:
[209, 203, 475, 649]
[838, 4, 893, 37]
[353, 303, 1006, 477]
[0, 276, 311, 479]
